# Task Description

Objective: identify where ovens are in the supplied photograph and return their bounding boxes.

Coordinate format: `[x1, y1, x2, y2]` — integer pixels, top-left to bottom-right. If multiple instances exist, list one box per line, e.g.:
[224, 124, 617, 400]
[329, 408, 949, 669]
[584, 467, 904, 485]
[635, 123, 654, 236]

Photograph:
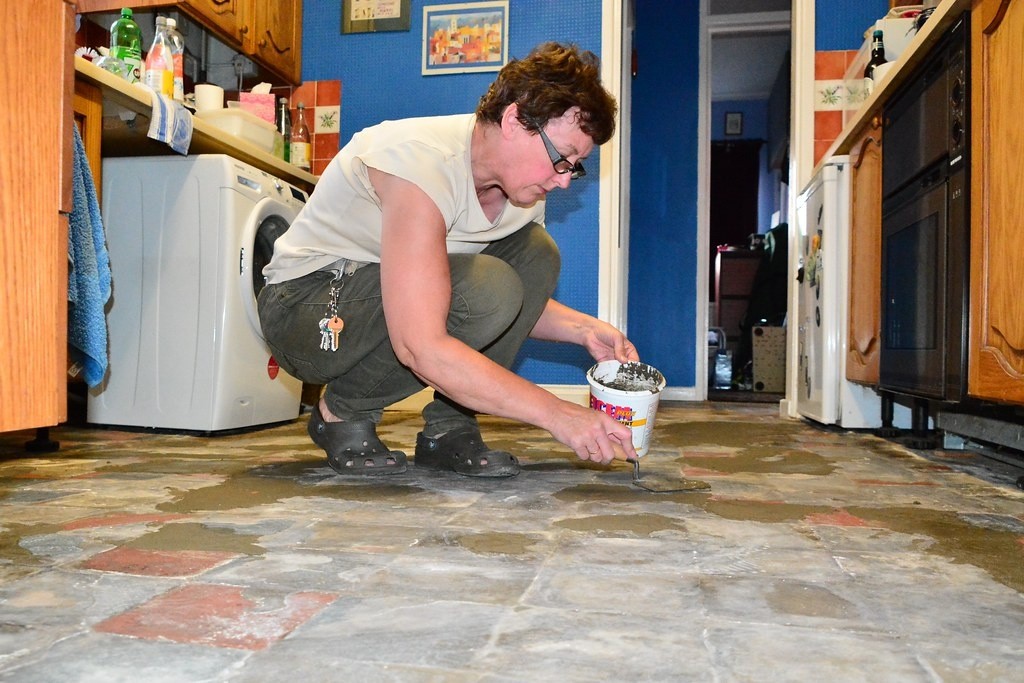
[881, 8, 973, 406]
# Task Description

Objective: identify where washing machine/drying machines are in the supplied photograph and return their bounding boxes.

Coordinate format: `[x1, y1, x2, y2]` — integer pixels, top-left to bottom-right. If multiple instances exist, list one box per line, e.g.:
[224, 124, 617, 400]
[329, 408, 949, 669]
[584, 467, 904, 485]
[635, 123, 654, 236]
[87, 152, 305, 433]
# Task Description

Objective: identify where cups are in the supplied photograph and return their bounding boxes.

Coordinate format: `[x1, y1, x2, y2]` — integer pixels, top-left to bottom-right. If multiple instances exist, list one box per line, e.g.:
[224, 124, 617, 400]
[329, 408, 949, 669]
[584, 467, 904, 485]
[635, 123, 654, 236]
[194, 84, 225, 111]
[586, 360, 667, 458]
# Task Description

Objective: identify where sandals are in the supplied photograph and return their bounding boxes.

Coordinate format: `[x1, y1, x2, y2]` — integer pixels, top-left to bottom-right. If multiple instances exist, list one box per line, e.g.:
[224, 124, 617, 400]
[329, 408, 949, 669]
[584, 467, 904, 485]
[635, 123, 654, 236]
[307, 399, 407, 475]
[414, 427, 519, 476]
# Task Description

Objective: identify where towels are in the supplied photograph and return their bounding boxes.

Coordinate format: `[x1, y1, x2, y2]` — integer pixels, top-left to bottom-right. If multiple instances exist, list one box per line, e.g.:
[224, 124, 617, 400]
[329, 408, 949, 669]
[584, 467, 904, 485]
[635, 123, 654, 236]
[68, 119, 115, 389]
[132, 81, 195, 156]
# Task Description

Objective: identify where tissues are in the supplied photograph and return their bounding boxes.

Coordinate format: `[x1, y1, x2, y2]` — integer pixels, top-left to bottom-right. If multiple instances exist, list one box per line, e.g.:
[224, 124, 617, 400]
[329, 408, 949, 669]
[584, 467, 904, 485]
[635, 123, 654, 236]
[240, 82, 276, 125]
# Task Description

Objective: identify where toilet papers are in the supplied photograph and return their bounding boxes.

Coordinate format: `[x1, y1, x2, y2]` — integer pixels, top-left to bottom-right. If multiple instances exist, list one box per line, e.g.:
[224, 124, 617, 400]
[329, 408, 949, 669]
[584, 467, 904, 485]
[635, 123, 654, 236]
[195, 84, 225, 114]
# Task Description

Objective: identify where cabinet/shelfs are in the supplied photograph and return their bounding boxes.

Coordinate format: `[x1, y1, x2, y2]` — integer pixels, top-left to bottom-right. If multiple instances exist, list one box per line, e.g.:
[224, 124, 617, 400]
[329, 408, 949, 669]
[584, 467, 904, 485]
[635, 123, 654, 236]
[969, 0, 1024, 405]
[0, 0, 319, 450]
[715, 251, 753, 351]
[845, 99, 882, 385]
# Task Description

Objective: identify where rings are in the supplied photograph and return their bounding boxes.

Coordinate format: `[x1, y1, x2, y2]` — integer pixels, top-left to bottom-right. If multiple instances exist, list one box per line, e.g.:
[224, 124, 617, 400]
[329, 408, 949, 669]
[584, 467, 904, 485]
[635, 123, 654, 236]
[589, 448, 602, 454]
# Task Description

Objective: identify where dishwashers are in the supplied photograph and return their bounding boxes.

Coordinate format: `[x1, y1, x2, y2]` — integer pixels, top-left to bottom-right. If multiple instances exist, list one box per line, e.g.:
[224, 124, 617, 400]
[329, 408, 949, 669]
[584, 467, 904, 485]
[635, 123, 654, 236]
[794, 156, 934, 433]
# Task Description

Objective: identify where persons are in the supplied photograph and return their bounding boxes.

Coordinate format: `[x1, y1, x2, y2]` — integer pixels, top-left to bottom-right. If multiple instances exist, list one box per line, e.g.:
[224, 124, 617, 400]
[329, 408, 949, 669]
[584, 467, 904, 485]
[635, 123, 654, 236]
[258, 42, 640, 476]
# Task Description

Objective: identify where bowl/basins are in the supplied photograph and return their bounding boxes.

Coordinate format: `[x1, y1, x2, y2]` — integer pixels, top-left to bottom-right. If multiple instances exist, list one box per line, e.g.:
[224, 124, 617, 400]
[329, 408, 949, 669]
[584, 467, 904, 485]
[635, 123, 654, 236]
[872, 60, 896, 83]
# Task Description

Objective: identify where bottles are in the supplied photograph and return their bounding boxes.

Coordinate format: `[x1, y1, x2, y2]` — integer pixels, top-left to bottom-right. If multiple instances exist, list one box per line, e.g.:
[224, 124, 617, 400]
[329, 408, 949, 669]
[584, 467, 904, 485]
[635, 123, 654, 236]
[165, 18, 185, 102]
[863, 30, 888, 100]
[144, 18, 175, 101]
[109, 8, 142, 85]
[275, 97, 292, 164]
[288, 101, 311, 173]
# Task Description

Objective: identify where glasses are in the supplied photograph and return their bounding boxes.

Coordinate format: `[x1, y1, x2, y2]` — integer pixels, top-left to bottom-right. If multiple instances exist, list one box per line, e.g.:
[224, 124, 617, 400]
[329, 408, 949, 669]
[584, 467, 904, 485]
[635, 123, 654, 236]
[527, 110, 586, 180]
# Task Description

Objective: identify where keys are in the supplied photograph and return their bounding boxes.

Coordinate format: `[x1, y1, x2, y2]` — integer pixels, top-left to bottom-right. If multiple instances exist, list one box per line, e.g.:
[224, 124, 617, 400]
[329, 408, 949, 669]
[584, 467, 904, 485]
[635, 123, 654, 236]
[318, 316, 344, 352]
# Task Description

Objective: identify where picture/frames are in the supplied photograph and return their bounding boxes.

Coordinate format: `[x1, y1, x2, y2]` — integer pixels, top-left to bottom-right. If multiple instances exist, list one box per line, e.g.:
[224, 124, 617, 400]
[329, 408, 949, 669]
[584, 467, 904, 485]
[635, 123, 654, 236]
[725, 112, 742, 135]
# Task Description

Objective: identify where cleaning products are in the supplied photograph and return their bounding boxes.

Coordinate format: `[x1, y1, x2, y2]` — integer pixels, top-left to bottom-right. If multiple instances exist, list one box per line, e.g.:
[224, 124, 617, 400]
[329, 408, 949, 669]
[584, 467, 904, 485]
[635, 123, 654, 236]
[109, 7, 142, 84]
